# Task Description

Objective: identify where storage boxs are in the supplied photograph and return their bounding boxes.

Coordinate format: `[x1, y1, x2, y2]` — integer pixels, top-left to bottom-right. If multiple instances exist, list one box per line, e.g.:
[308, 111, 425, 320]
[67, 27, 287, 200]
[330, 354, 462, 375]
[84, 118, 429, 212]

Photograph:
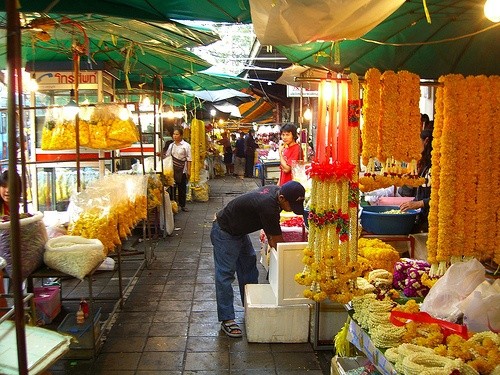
[57, 313, 100, 349]
[244, 242, 352, 344]
[31, 287, 61, 323]
[330, 355, 378, 375]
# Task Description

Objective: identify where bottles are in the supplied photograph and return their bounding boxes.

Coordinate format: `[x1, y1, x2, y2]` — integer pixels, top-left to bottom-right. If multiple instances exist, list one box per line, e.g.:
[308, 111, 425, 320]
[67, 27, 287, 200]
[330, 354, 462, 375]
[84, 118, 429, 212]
[75, 305, 85, 325]
[80, 296, 90, 319]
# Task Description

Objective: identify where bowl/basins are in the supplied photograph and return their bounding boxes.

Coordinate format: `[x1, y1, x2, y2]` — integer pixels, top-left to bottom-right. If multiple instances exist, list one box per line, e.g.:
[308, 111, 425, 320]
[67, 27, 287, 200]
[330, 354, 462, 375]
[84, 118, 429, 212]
[360, 205, 421, 235]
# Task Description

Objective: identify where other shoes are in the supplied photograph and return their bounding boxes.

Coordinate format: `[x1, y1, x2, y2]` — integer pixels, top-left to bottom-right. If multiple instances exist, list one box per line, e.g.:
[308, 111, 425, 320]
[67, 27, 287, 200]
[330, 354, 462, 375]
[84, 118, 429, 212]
[182, 207, 189, 212]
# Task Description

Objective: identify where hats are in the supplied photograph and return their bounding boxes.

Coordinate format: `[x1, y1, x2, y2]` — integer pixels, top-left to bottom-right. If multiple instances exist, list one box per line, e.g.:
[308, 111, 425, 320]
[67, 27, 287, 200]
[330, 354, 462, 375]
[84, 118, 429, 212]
[248, 128, 256, 132]
[282, 181, 305, 215]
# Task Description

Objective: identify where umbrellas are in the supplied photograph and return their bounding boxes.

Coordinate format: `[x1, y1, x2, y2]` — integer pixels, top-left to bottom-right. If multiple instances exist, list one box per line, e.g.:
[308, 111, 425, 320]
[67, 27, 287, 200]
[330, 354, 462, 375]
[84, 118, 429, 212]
[0, 0, 253, 375]
[274, 0, 500, 88]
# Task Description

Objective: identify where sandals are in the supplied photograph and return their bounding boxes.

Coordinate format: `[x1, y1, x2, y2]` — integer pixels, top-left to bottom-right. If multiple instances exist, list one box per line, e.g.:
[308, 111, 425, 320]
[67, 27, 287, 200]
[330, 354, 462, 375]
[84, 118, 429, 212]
[220, 320, 244, 338]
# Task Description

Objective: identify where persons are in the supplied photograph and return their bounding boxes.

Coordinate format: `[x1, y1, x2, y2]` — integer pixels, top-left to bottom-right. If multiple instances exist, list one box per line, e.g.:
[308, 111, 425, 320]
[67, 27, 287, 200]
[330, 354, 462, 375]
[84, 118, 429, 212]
[217, 128, 260, 177]
[210, 182, 305, 337]
[0, 170, 22, 220]
[156, 128, 192, 212]
[279, 123, 304, 186]
[398, 113, 434, 234]
